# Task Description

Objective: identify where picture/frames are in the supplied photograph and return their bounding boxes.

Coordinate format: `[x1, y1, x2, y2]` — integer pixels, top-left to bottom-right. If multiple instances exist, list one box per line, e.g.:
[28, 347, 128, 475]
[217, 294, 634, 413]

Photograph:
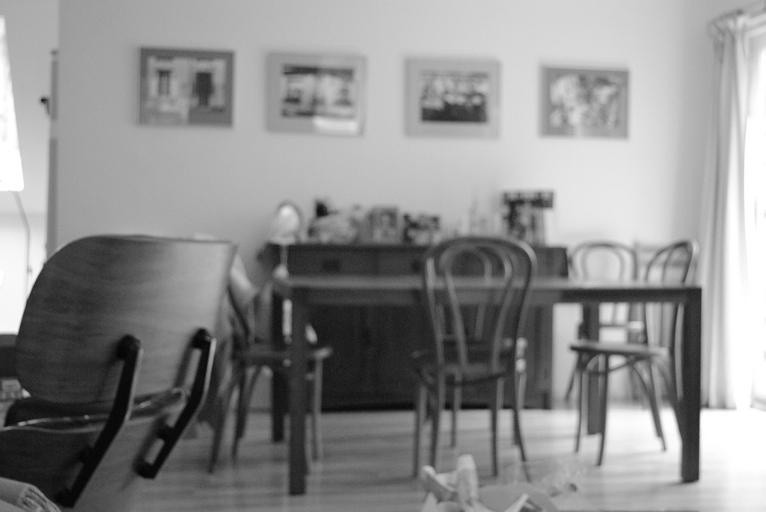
[137, 47, 238, 129]
[266, 52, 369, 139]
[404, 57, 504, 140]
[537, 65, 632, 140]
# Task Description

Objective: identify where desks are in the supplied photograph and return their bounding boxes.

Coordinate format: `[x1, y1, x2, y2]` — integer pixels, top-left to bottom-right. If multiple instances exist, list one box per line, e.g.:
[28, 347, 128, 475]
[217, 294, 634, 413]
[271, 272, 701, 493]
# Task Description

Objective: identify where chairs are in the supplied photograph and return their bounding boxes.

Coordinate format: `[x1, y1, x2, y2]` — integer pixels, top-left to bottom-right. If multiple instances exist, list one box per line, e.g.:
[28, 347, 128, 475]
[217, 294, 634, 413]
[0, 233, 238, 512]
[573, 241, 696, 466]
[407, 236, 537, 480]
[569, 245, 650, 403]
[211, 258, 333, 476]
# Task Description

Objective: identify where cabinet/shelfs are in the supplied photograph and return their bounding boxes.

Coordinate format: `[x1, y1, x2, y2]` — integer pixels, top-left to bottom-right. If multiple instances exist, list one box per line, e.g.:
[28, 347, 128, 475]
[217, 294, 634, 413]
[255, 244, 572, 412]
